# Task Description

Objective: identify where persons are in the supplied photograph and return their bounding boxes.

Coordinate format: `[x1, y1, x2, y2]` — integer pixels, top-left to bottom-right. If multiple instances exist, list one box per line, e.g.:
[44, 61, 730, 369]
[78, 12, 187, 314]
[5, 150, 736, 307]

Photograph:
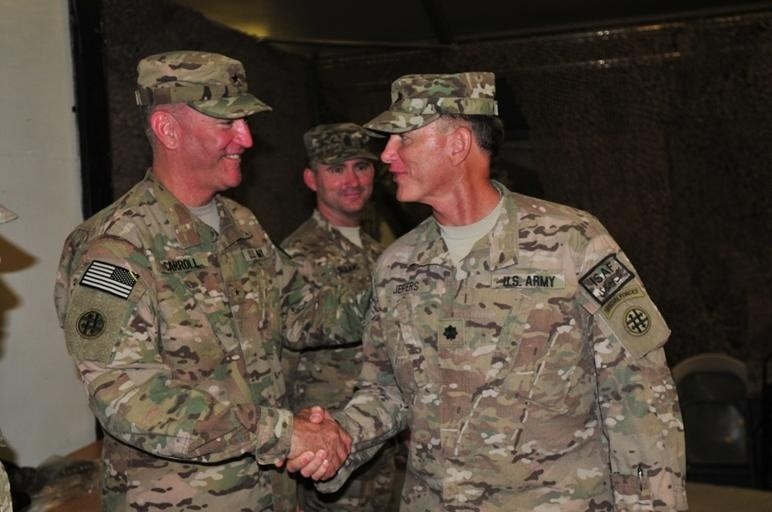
[54, 51, 353, 512]
[280, 124, 410, 511]
[275, 72, 686, 511]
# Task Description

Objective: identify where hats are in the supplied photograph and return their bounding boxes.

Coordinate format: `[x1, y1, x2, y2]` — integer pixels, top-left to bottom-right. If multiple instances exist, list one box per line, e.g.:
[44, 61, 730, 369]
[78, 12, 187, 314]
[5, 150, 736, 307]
[361, 71, 500, 138]
[303, 122, 379, 165]
[134, 50, 273, 119]
